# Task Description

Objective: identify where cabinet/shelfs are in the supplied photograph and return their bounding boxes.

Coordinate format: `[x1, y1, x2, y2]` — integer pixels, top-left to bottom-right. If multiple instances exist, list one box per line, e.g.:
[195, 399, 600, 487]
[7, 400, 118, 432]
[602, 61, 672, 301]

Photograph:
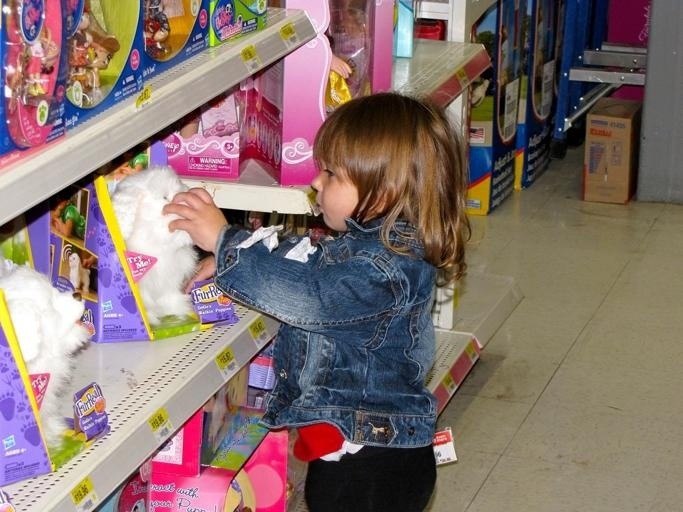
[2, 0, 494, 511]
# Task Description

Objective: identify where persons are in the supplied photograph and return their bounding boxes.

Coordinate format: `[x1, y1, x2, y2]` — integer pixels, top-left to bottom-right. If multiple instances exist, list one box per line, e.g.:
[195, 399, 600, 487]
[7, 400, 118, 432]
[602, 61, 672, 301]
[5, 24, 56, 114]
[143, 0, 177, 57]
[67, 0, 119, 106]
[164, 87, 472, 512]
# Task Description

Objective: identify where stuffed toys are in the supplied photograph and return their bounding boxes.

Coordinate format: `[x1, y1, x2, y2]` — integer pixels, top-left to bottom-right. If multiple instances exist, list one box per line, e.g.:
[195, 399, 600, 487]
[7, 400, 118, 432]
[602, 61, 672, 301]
[109, 166, 193, 325]
[0, 257, 95, 454]
